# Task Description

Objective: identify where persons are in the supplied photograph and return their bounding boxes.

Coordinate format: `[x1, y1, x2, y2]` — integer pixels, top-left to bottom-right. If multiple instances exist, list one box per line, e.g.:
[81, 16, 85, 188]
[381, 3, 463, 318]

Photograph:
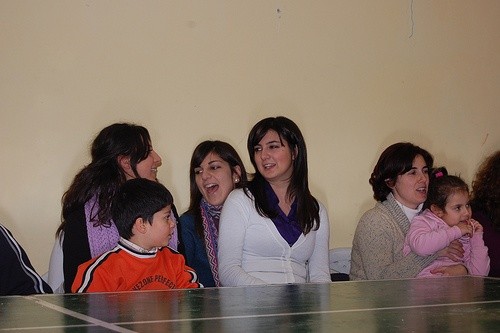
[404, 175, 490, 279]
[48, 123, 187, 292]
[349, 141, 468, 281]
[469, 150, 500, 277]
[178, 140, 248, 287]
[70, 178, 204, 293]
[0, 223, 53, 296]
[217, 116, 332, 286]
